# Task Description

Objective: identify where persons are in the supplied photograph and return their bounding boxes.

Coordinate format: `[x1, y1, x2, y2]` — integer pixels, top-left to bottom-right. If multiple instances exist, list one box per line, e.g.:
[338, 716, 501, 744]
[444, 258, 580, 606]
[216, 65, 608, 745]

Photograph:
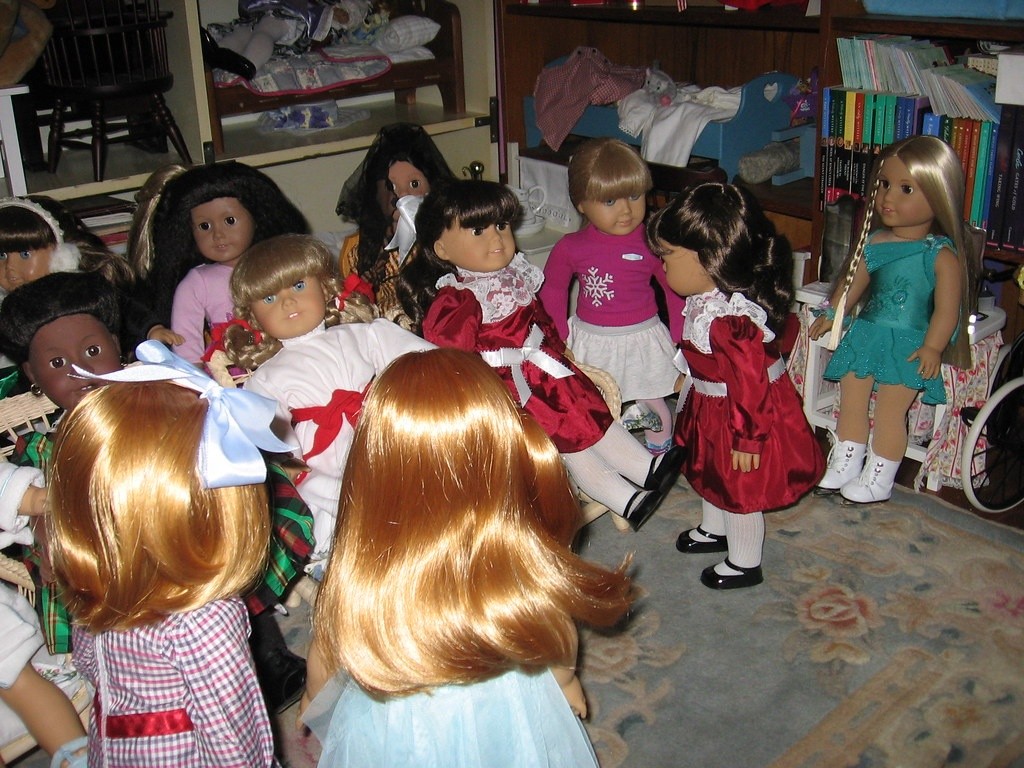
[406, 179, 687, 531]
[806, 124, 976, 516]
[0, 193, 315, 768]
[138, 155, 316, 378]
[295, 345, 644, 767]
[535, 138, 686, 453]
[196, 0, 400, 79]
[37, 338, 300, 767]
[645, 180, 829, 589]
[328, 118, 459, 334]
[222, 233, 442, 570]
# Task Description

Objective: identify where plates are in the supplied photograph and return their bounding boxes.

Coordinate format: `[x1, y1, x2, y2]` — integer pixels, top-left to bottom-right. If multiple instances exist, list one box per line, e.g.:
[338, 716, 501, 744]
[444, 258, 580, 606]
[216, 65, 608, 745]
[512, 214, 546, 238]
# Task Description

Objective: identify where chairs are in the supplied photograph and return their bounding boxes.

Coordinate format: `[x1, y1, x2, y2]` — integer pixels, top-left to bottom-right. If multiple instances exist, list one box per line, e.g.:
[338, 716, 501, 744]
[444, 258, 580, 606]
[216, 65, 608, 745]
[39, 1, 194, 182]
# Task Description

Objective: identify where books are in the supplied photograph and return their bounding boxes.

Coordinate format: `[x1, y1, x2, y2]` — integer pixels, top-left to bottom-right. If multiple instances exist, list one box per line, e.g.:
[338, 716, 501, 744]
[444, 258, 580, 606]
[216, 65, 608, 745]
[815, 34, 1024, 251]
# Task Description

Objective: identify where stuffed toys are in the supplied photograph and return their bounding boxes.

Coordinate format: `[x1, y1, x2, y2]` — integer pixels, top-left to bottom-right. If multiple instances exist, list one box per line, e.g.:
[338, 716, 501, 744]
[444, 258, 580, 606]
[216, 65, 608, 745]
[640, 68, 677, 107]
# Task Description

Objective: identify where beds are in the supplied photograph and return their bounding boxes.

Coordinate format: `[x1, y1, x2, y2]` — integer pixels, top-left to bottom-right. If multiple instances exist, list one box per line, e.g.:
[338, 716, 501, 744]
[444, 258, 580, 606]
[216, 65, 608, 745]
[203, 0, 465, 157]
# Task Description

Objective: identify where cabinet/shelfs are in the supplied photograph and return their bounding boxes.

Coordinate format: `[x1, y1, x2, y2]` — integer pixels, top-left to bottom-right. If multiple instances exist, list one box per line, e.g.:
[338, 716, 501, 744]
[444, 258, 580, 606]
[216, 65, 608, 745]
[494, 1, 1024, 285]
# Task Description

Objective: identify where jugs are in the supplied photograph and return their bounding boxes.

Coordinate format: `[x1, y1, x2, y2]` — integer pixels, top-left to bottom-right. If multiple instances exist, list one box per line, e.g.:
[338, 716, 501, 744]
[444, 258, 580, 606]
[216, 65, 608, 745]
[504, 183, 548, 226]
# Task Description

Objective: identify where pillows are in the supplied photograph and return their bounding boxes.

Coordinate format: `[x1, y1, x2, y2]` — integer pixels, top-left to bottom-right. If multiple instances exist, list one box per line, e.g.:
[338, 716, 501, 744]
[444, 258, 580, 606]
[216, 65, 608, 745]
[376, 14, 442, 53]
